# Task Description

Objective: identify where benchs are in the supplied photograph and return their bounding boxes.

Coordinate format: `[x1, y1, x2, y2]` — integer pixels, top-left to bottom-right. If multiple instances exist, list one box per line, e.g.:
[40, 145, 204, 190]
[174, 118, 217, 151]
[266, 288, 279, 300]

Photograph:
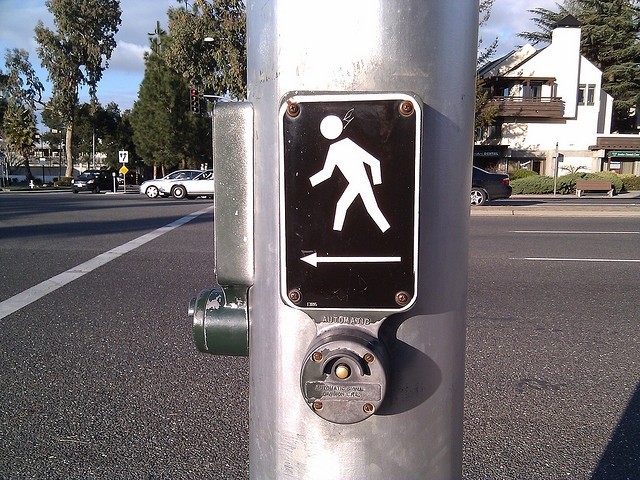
[576, 179, 613, 198]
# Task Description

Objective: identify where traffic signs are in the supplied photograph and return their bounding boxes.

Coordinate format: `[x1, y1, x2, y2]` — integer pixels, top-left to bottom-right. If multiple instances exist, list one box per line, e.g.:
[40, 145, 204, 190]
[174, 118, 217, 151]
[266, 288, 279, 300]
[279, 93, 422, 312]
[119, 151, 128, 163]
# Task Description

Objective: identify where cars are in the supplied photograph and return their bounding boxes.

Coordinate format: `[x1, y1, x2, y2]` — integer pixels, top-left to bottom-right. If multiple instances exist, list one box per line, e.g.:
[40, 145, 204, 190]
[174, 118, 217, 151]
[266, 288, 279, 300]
[71, 170, 119, 194]
[117, 171, 144, 185]
[470, 165, 512, 207]
[139, 169, 203, 200]
[158, 170, 214, 200]
